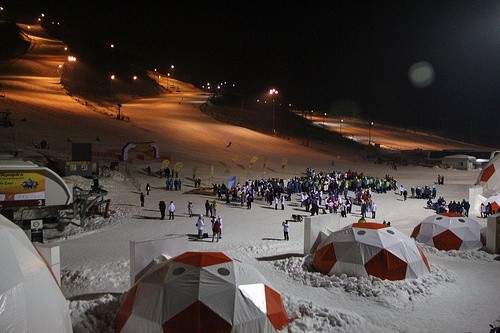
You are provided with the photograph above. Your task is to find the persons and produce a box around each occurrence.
[282,220,289,240]
[41,139,47,149]
[480,202,492,219]
[147,166,182,191]
[186,199,223,242]
[438,174,444,185]
[211,168,398,219]
[146,183,150,195]
[400,184,470,217]
[140,192,145,207]
[159,200,176,220]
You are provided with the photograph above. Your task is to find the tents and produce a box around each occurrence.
[476,152,500,198]
[114,252,289,333]
[311,222,431,281]
[0,213,74,333]
[410,212,482,251]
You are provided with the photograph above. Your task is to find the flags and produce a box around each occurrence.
[160,154,289,180]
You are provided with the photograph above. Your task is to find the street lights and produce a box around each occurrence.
[368,122,373,145]
[310,110,313,121]
[269,88,279,135]
[339,118,343,134]
[322,112,327,129]
